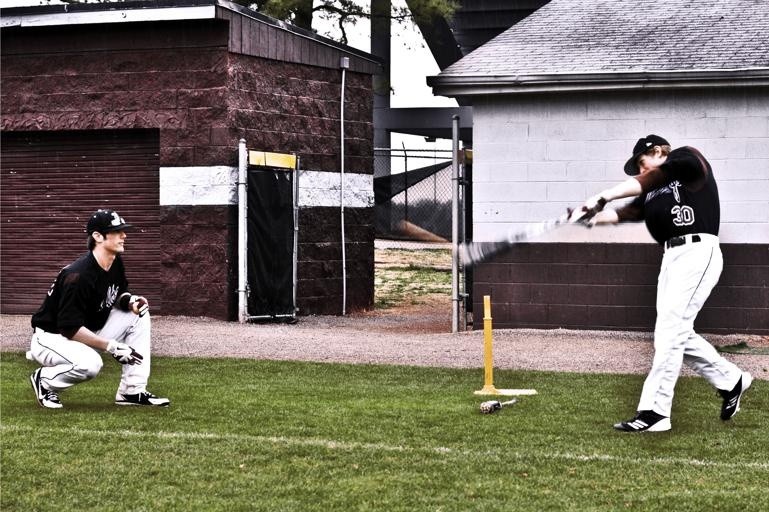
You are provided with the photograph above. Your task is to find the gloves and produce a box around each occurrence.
[106,340,144,365]
[128,296,149,318]
[567,194,607,230]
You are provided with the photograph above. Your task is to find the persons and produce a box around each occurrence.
[568,134,755,433]
[29,208,170,409]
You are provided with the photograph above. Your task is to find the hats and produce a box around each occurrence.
[87,210,133,235]
[624,134,670,176]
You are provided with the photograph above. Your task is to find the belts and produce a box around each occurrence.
[667,236,701,249]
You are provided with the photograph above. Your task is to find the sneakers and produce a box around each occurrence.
[29,366,64,409]
[115,391,170,406]
[613,410,672,432]
[718,372,752,420]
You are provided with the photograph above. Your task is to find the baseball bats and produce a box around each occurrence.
[479,397,515,414]
[454,202,583,269]
